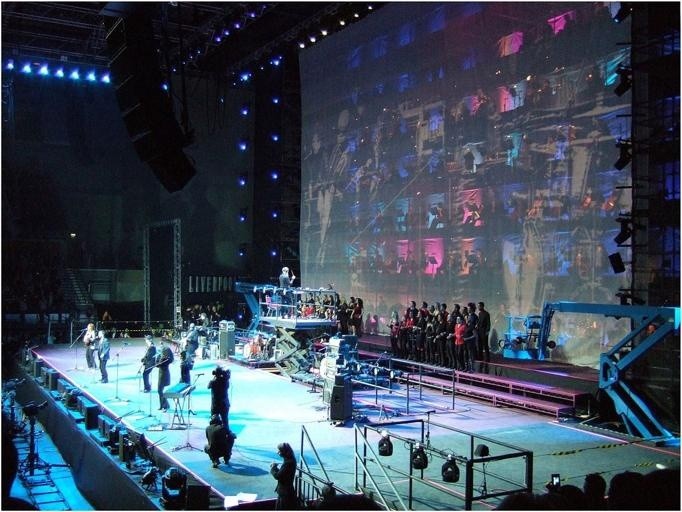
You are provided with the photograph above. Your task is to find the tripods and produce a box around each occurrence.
[65,336,86,373]
[136,370,158,420]
[172,394,204,453]
[104,361,131,404]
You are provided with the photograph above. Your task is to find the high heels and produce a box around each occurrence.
[187,408,197,417]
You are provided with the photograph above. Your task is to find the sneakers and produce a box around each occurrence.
[157,406,171,414]
[98,377,108,384]
[210,458,231,470]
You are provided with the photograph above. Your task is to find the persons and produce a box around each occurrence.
[1,267,679,511]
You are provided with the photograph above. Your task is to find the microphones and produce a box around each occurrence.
[80,328,89,333]
[123,341,132,347]
[194,372,205,377]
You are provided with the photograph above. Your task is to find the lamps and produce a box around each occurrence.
[608,3,649,308]
[355,363,404,382]
[373,428,462,482]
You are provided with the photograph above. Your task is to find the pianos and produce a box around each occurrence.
[163,382,196,398]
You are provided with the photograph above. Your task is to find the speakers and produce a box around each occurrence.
[331,385,354,419]
[96,12,198,194]
[218,330,235,360]
